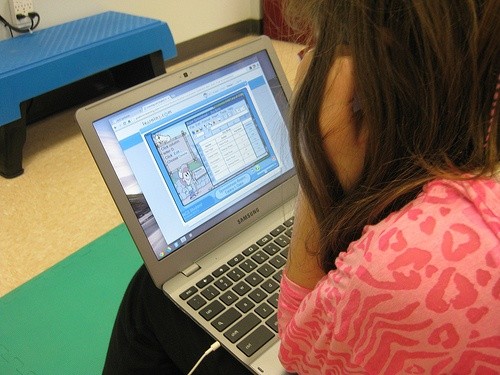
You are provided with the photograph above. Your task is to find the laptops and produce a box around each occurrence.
[74,34,301,375]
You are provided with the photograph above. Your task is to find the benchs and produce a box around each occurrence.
[0,10,179,178]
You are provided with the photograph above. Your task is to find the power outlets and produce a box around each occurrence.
[8,0,34,29]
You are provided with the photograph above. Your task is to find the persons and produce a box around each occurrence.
[101,0,500,375]
